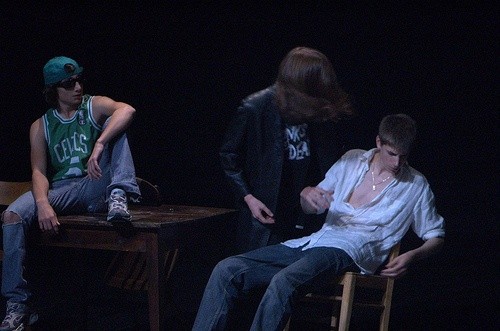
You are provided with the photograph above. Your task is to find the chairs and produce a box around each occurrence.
[278,241,401,331]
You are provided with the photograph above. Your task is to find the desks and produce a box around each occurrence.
[32,206,239,331]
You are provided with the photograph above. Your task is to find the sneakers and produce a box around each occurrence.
[0,301,38,331]
[105,191,132,221]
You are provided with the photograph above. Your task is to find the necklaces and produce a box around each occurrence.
[371,160,399,191]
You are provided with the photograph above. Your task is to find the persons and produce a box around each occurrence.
[222,47,355,254]
[192,114,445,331]
[0,55,142,331]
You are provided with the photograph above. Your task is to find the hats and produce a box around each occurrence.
[43,56,84,89]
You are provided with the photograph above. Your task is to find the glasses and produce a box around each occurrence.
[54,75,85,90]
